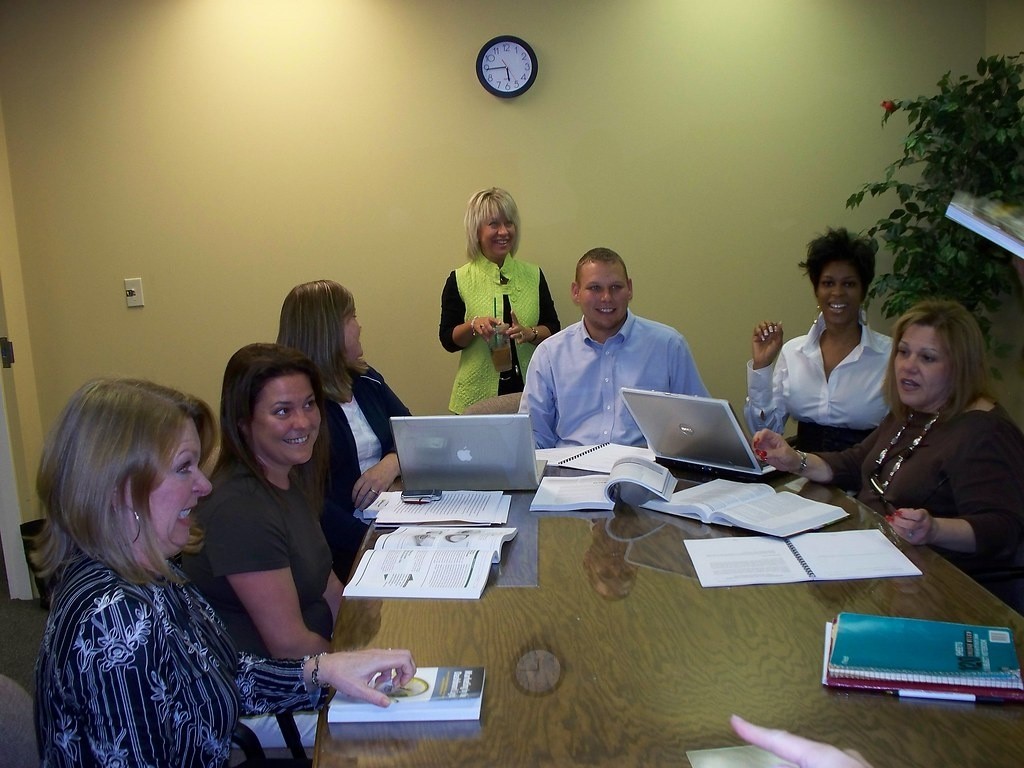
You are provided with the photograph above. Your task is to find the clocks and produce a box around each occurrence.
[475,35,538,99]
[510,643,565,697]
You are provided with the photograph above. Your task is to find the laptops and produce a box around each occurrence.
[389,414,548,491]
[619,386,778,482]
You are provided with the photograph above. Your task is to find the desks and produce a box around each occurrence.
[311,466,1024,768]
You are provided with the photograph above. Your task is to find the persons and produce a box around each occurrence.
[273,278,416,581]
[437,188,561,419]
[745,226,897,453]
[517,245,714,456]
[581,515,644,600]
[185,341,346,749]
[729,713,873,768]
[752,299,1023,604]
[19,380,414,768]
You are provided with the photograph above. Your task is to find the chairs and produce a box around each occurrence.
[0,675,44,768]
[463,392,521,415]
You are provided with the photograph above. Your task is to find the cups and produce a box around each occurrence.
[486,323,512,373]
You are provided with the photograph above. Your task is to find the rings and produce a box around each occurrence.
[370,488,379,494]
[519,332,523,336]
[480,324,484,329]
[908,531,913,539]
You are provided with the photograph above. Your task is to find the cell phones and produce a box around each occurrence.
[401,490,442,501]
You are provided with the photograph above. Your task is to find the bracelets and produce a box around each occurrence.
[311,652,332,688]
[529,326,538,342]
[793,449,807,475]
[471,315,480,336]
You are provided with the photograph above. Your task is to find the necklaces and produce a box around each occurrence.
[868,401,944,500]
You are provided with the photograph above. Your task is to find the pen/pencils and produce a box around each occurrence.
[886,688,1003,702]
[880,495,896,515]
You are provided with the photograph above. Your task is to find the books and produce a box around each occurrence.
[682,526,926,589]
[535,442,659,476]
[528,456,677,513]
[816,612,1023,703]
[637,477,851,539]
[327,660,487,723]
[341,524,518,599]
